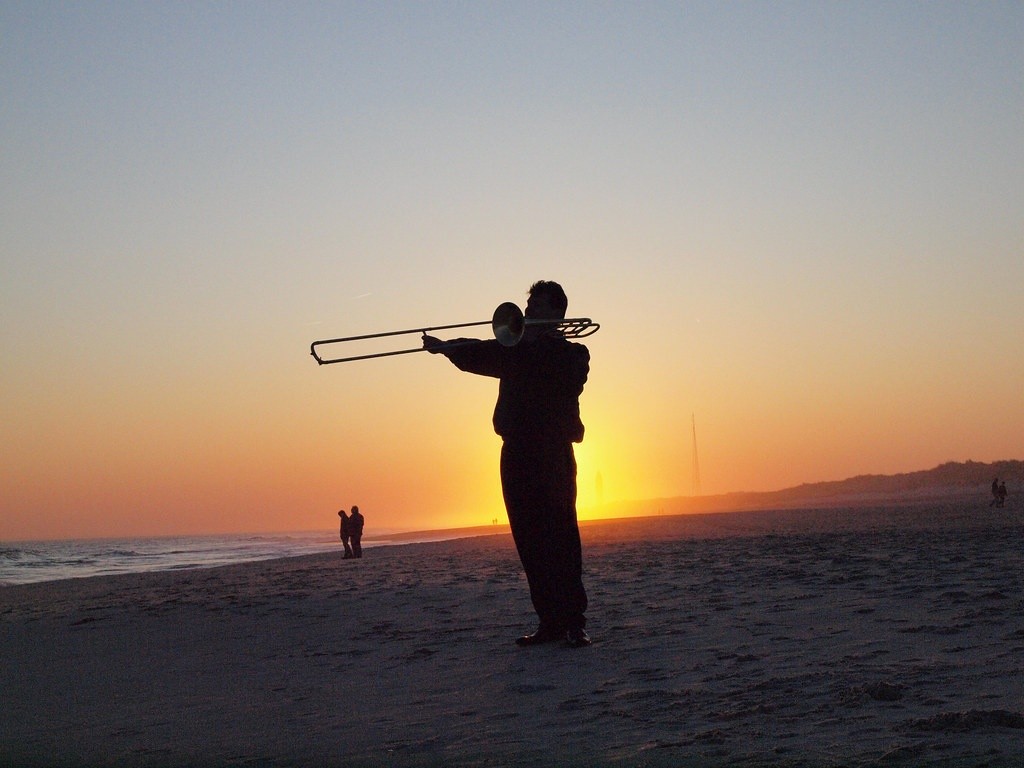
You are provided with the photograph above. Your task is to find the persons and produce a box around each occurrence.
[989,477,1008,509]
[338,506,365,559]
[422,280,591,646]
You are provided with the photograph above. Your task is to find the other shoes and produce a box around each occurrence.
[567,627,590,649]
[515,626,567,645]
[341,555,352,560]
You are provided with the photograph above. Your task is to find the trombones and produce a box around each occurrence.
[308,302,601,366]
